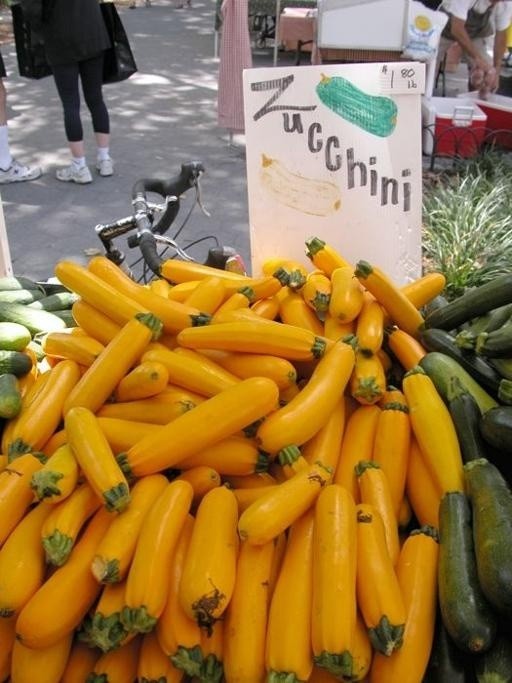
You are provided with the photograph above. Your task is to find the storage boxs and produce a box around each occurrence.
[425,89,512,160]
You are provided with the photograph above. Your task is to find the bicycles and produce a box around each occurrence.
[94,158,248,286]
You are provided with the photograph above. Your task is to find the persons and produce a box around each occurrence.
[9,0,137,184]
[435,0,512,92]
[0,50,42,183]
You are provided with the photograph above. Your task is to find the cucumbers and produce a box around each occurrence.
[261,159,340,216]
[316,77,397,137]
[0,236,512,683]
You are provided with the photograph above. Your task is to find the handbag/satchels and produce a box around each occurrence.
[99,2,137,83]
[440,5,493,40]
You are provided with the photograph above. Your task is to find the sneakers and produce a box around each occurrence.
[1,157,41,184]
[56,165,92,185]
[96,154,115,177]
